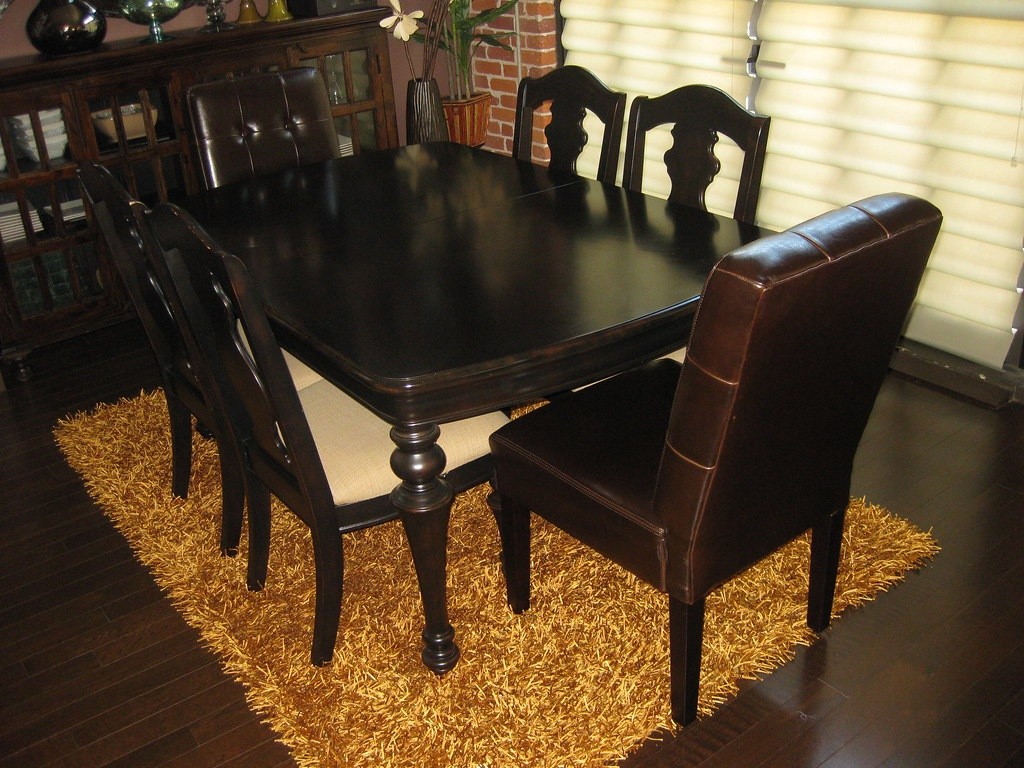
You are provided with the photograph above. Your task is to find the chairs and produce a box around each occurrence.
[78,67,942,726]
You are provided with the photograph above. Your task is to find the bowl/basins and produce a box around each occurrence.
[10,107,69,162]
[90,102,158,143]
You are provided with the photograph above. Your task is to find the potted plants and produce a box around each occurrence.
[410,0,521,149]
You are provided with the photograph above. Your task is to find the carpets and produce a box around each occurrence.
[52,386,942,768]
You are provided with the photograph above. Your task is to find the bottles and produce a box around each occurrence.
[25,0,107,58]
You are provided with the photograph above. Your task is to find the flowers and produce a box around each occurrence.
[380,0,453,80]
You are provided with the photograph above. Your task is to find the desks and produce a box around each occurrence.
[164,141,778,677]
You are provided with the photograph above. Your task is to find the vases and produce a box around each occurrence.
[407,78,448,145]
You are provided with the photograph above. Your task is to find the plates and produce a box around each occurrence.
[0,199,45,243]
[337,134,353,158]
[43,198,86,221]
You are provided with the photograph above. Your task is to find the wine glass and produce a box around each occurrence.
[117,0,185,45]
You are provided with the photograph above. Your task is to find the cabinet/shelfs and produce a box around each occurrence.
[0,9,399,382]
